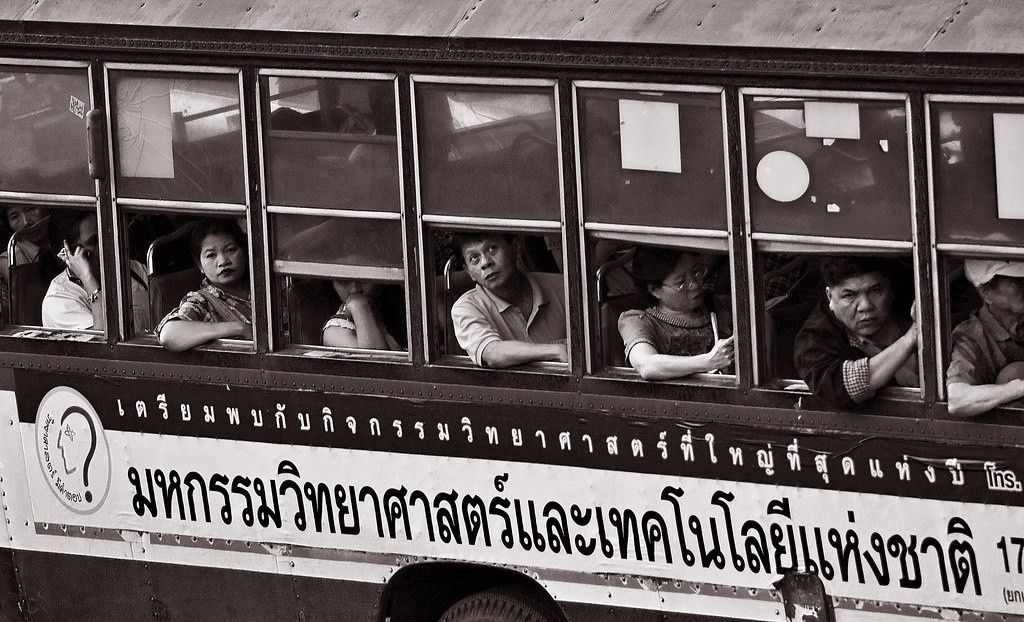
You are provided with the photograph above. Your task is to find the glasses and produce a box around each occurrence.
[659,266,707,292]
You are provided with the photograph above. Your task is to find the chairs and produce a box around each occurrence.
[0,101,981,378]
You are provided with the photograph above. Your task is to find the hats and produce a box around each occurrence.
[964,232,1024,288]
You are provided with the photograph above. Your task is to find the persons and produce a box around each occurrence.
[617,243,735,382]
[450,232,567,370]
[946,259,1024,418]
[319,239,408,352]
[793,255,918,407]
[153,218,253,353]
[0,202,159,340]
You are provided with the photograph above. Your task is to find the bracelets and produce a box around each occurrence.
[86,285,100,305]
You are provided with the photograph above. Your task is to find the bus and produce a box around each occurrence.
[0,0,1024,622]
